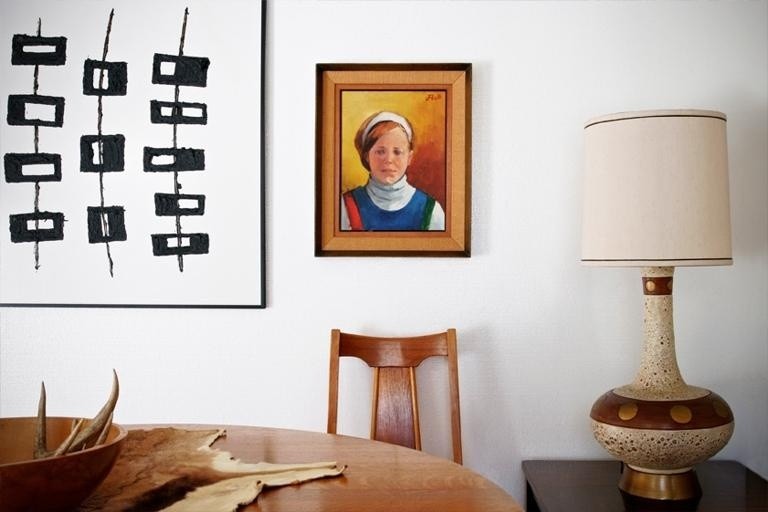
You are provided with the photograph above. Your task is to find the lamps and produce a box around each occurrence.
[578,109,737,510]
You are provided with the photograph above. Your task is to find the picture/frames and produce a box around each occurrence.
[0,0,275,313]
[311,60,472,260]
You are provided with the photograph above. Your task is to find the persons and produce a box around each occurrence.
[341,110,445,230]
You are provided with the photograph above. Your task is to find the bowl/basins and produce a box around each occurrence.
[0,417,131,511]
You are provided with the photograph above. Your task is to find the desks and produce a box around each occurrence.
[76,423,523,512]
[523,454,766,512]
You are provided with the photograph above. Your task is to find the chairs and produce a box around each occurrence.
[327,320,466,466]
